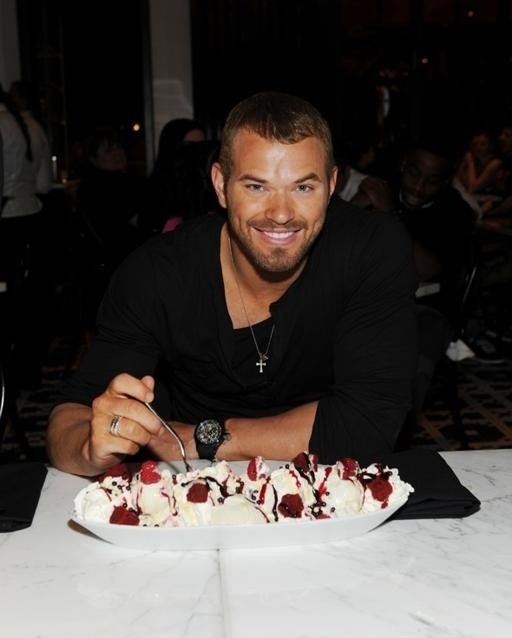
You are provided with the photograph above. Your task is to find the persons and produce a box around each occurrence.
[43,87,423,477]
[329,87,511,364]
[1,76,208,374]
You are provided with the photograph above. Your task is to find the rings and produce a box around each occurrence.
[110,416,124,433]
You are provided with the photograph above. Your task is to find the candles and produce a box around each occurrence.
[51,155,58,177]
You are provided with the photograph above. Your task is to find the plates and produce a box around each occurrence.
[69,459,410,551]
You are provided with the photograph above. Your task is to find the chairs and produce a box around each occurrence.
[393,262,481,450]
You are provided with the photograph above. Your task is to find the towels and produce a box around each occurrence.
[358,438,482,520]
[0,444,48,533]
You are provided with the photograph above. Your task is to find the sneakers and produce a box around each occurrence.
[468,336,505,365]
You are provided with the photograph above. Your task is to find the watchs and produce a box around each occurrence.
[193,415,234,461]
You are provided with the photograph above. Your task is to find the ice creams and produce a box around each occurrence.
[84,453,415,528]
